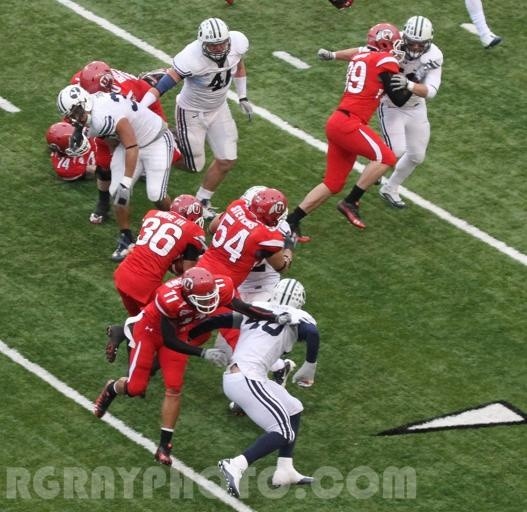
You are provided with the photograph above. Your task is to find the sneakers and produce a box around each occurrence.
[480,32,502,49]
[89,204,110,225]
[229,402,246,416]
[273,310,292,325]
[112,233,137,262]
[272,359,297,388]
[218,458,242,498]
[153,442,173,464]
[94,379,118,419]
[200,199,221,221]
[336,197,368,229]
[106,326,126,363]
[377,182,408,209]
[272,469,315,488]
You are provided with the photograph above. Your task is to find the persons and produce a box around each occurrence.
[461,1,503,52]
[45,14,446,502]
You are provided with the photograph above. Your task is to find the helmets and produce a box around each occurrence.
[197,18,231,61]
[367,23,406,63]
[403,16,434,61]
[47,60,115,158]
[170,194,205,229]
[270,278,306,310]
[182,267,220,314]
[240,185,289,230]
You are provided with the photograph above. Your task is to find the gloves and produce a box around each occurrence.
[292,359,317,388]
[390,74,408,92]
[282,231,298,254]
[239,97,253,123]
[201,347,232,368]
[317,48,336,62]
